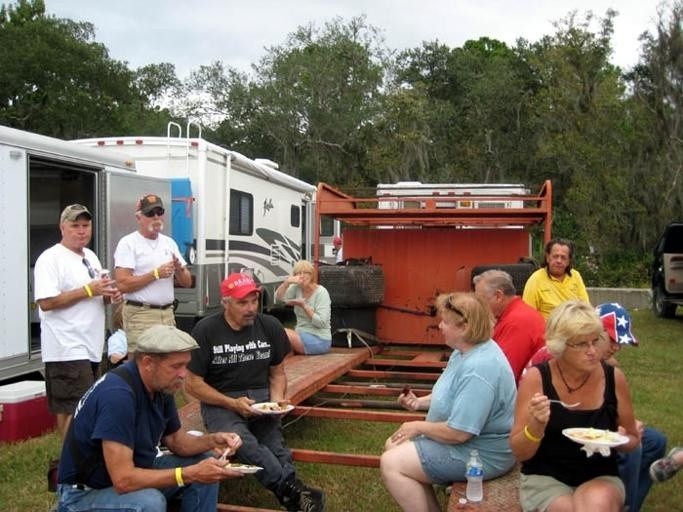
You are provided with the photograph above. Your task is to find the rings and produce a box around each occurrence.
[397,433,400,438]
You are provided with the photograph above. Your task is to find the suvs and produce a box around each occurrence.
[650,222,683,320]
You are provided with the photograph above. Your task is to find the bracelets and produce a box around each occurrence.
[174,466,184,489]
[83,284,93,297]
[524,424,542,443]
[153,268,160,281]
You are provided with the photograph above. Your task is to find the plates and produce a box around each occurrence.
[561,427,630,448]
[250,402,295,414]
[222,463,265,473]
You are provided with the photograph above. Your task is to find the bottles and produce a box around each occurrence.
[464,449,483,503]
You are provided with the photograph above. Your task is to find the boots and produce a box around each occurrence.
[269,472,326,512]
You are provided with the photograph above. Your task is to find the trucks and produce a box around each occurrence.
[62,120,344,344]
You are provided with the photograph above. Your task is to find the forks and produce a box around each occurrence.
[548,399,581,408]
[218,436,240,461]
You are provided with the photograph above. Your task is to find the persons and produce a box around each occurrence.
[379,291,519,512]
[334,238,344,266]
[517,302,666,512]
[508,302,640,512]
[113,194,191,353]
[106,303,129,367]
[523,238,589,325]
[184,272,327,512]
[471,268,547,387]
[33,202,122,442]
[56,324,243,512]
[276,259,331,355]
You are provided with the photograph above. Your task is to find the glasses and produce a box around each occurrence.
[83,258,95,279]
[445,296,467,323]
[565,333,607,350]
[144,208,165,217]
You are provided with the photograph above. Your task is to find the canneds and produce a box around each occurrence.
[100,271,112,289]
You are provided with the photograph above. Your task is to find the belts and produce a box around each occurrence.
[127,300,172,310]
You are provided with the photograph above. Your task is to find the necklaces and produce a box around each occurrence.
[556,356,592,397]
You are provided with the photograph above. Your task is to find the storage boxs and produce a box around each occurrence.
[0,380,58,443]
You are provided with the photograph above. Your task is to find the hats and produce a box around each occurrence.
[137,195,163,213]
[60,204,92,224]
[136,325,200,354]
[594,302,640,347]
[221,273,262,299]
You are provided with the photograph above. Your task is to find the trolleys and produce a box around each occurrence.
[170,176,197,266]
